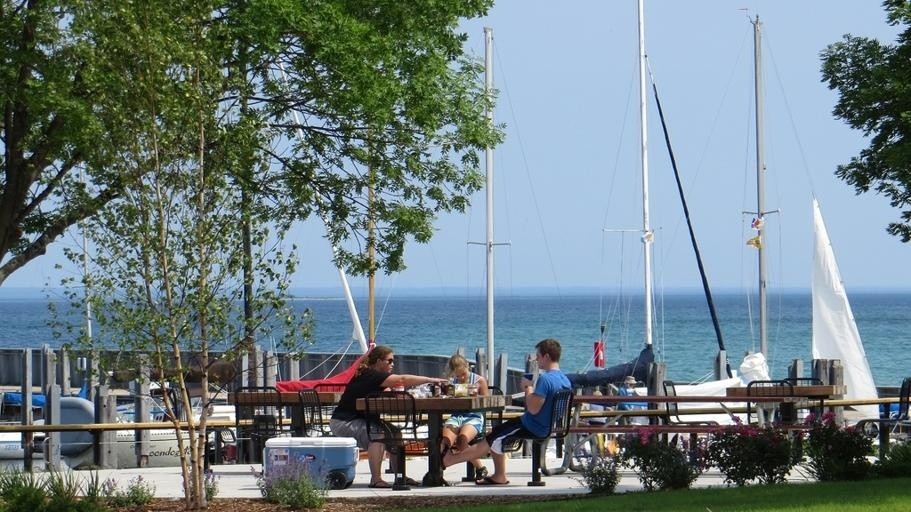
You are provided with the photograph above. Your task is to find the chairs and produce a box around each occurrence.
[785,378,824,418]
[856,377,911,465]
[235,386,296,475]
[299,389,367,453]
[747,380,807,466]
[502,389,575,486]
[663,380,722,465]
[310,383,348,434]
[171,383,236,472]
[462,386,505,482]
[365,391,430,490]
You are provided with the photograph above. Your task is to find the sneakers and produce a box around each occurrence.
[476,466,488,480]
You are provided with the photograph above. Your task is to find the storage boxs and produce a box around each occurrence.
[262,437,360,489]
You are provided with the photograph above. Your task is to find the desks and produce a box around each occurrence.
[356,394,506,486]
[726,385,847,462]
[227,391,344,438]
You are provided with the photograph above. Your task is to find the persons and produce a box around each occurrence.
[329,346,449,488]
[442,356,489,485]
[588,375,644,424]
[475,338,571,486]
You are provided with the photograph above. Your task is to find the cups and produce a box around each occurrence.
[523,373,533,385]
[467,385,478,397]
[394,383,404,393]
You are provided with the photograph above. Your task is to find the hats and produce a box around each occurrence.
[625,376,637,384]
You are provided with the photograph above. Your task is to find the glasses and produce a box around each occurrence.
[382,358,394,364]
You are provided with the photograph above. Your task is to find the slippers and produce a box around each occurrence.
[406,477,421,486]
[368,478,390,487]
[475,476,509,485]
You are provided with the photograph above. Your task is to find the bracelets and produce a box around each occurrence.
[427,378,430,384]
